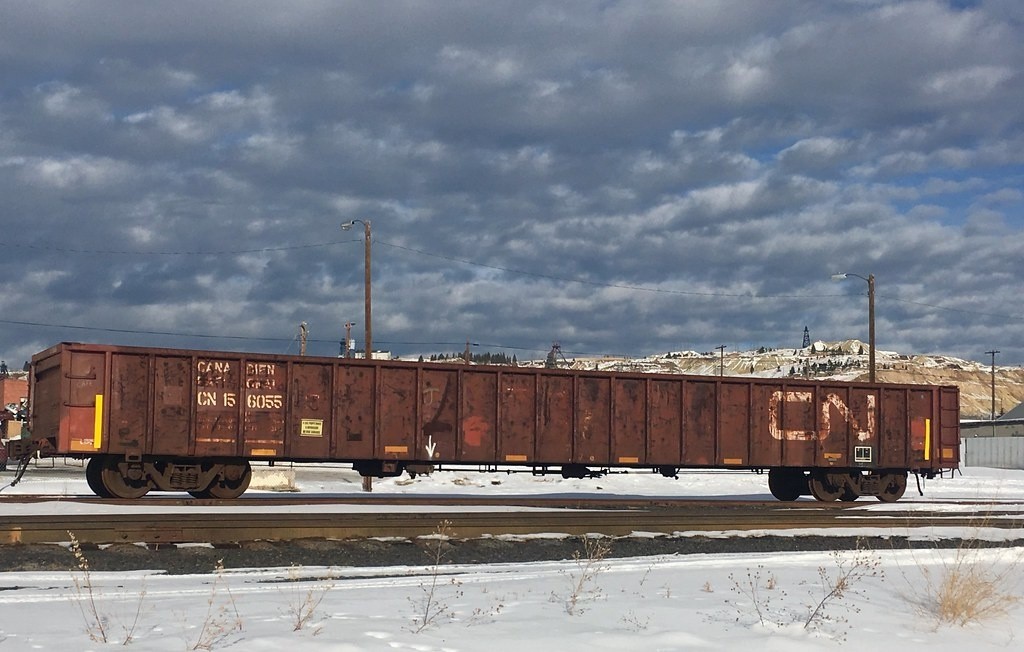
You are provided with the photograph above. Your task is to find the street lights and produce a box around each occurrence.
[832,272,876,383]
[340,216,373,359]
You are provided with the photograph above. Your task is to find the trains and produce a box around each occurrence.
[0,340,960,502]
